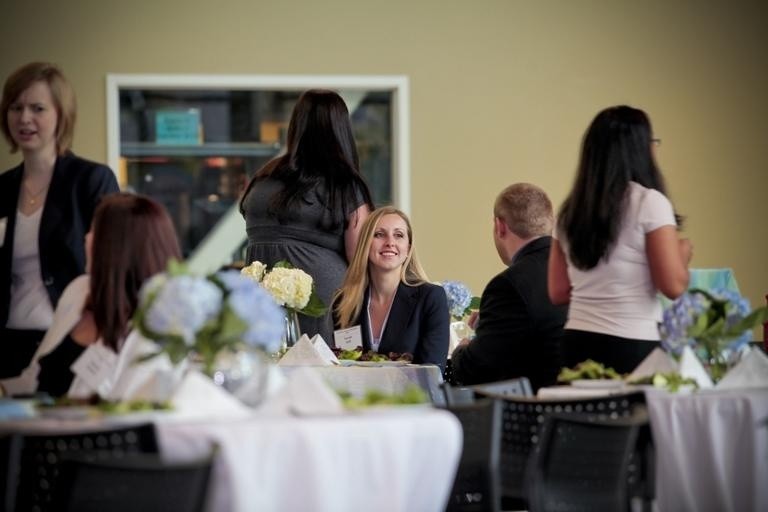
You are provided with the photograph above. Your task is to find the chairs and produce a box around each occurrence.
[533,412,647,512]
[3,420,160,509]
[58,439,217,512]
[434,403,513,512]
[473,388,656,508]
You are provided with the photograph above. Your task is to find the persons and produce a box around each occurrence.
[238,88,373,353]
[549,105,695,373]
[2,192,184,398]
[333,206,450,385]
[2,59,122,397]
[450,182,574,393]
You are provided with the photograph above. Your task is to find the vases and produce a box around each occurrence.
[282,305,300,344]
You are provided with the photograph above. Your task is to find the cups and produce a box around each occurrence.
[676,214,688,240]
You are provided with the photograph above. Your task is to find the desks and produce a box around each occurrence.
[293,354,441,399]
[539,384,767,510]
[1,390,461,511]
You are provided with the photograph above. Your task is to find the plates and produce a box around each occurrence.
[336,359,355,367]
[35,404,95,420]
[357,359,407,367]
[572,379,626,389]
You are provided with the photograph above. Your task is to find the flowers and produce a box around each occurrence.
[433,281,471,317]
[239,259,321,320]
[659,284,767,354]
[133,262,283,373]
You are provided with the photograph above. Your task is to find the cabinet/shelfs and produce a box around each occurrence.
[108,73,413,261]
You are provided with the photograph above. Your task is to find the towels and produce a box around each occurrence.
[106,330,335,406]
[630,345,764,386]
